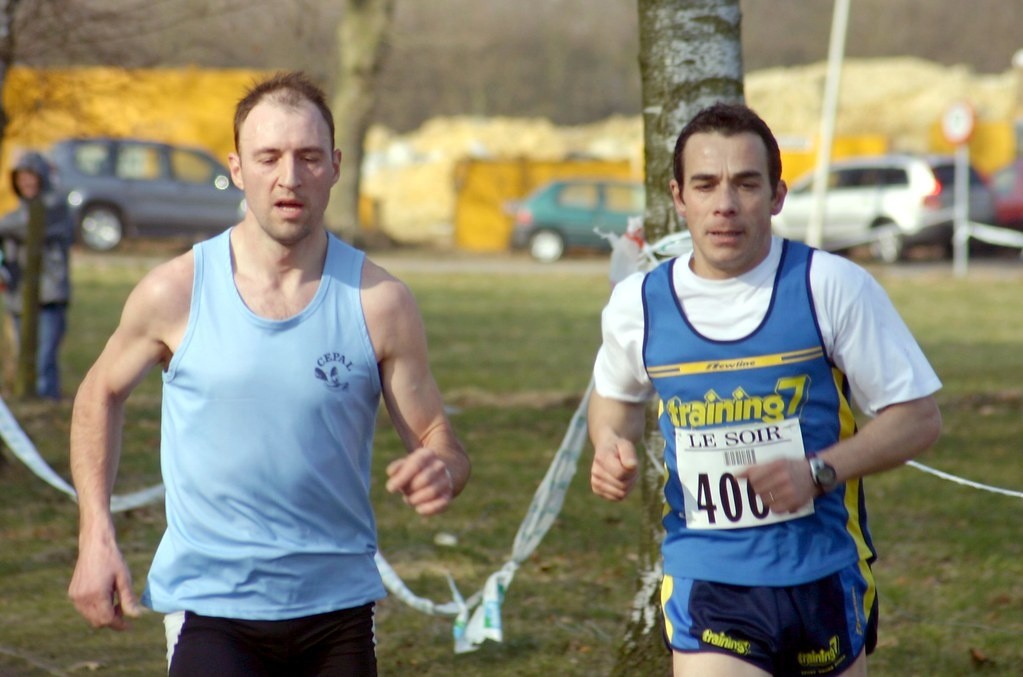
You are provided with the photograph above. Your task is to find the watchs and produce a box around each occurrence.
[805,451,837,494]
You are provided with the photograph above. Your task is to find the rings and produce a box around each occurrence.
[769,491,775,503]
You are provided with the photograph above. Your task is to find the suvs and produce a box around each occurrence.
[43,133,249,254]
[770,153,994,265]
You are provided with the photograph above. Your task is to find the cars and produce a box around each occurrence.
[941,157,1023,256]
[509,177,645,264]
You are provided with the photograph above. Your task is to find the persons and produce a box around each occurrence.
[0,153,73,401]
[589,101,943,677]
[69,71,471,676]
